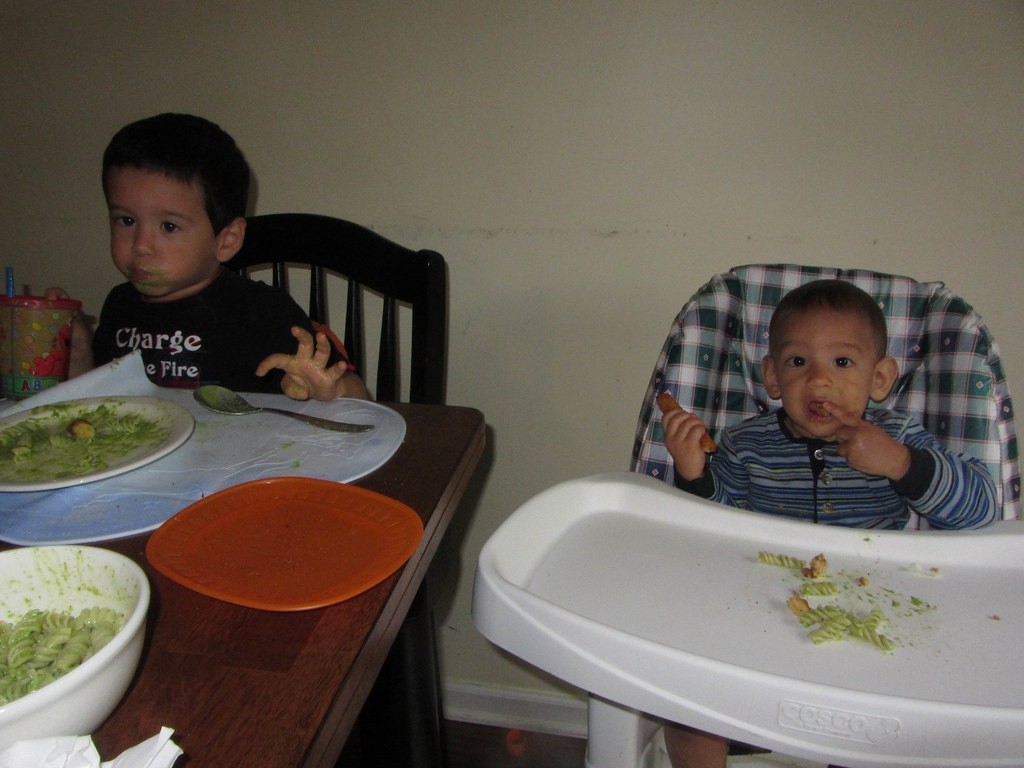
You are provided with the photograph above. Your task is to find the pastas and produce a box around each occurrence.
[759,553,893,650]
[0,406,166,469]
[0,609,118,711]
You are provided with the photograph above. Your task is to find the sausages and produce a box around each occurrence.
[657,395,716,452]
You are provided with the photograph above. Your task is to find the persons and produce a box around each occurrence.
[46,112,372,403]
[659,277,999,767]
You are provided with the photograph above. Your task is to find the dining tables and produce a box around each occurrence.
[0,388,487,768]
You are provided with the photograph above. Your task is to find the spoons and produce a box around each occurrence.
[192,385,375,434]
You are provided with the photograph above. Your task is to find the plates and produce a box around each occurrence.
[0,394,194,492]
[145,480,422,612]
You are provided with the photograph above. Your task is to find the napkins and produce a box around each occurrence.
[0,726,184,768]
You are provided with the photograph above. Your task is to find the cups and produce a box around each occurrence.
[0,294,83,401]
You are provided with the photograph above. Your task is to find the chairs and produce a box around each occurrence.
[221,213,447,408]
[472,262,1024,767]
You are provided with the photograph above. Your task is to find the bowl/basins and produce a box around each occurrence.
[0,545,151,752]
